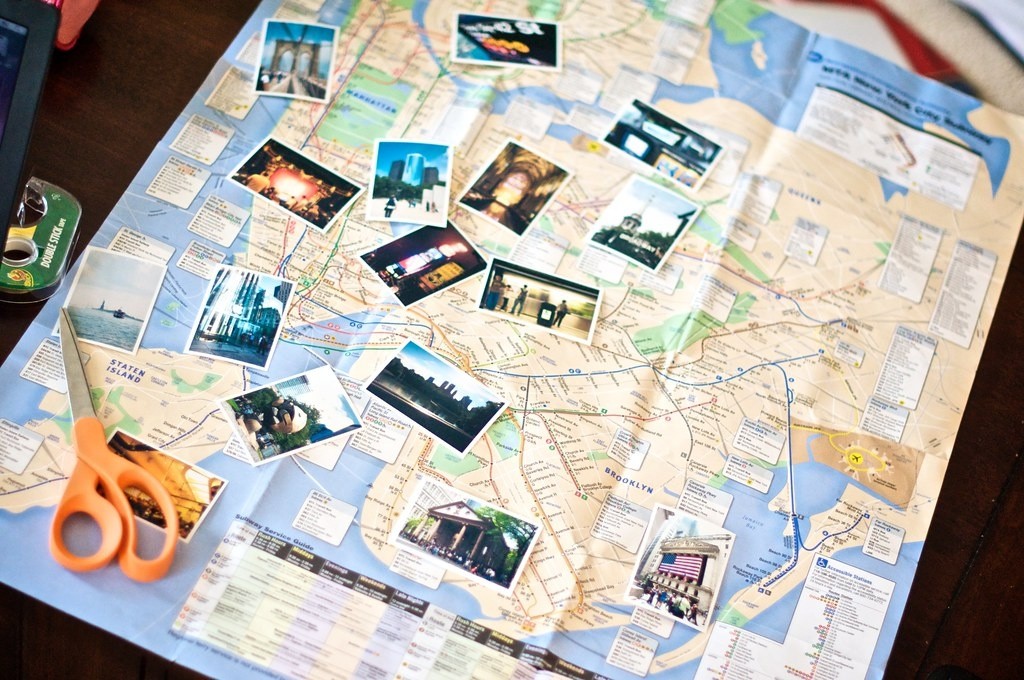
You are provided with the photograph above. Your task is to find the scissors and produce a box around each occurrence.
[50,306,178,583]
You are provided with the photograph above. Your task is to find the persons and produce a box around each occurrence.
[277,72,282,83]
[399,530,495,581]
[244,169,319,222]
[648,588,698,625]
[486,274,506,311]
[553,300,567,327]
[384,194,396,216]
[509,284,529,317]
[261,71,270,92]
[501,284,513,311]
